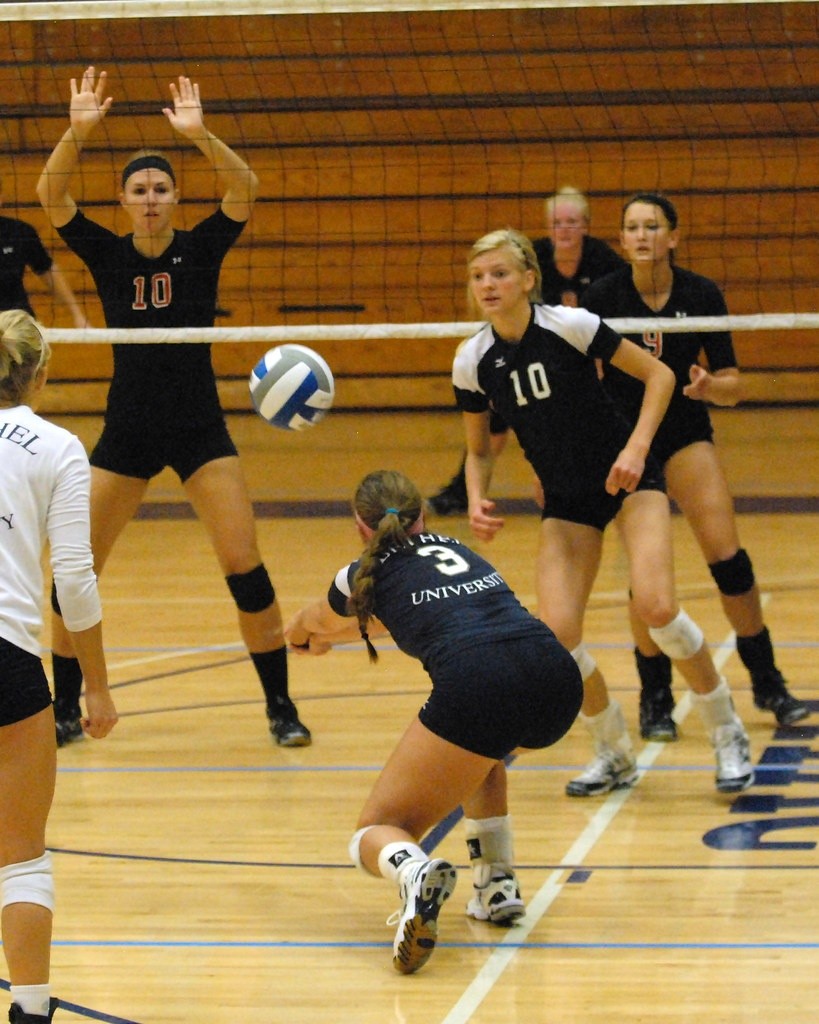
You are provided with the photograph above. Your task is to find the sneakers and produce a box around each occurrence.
[703,713,755,794]
[53,697,85,747]
[464,873,527,925]
[266,694,312,748]
[392,856,458,974]
[638,685,677,741]
[752,670,809,724]
[564,744,642,798]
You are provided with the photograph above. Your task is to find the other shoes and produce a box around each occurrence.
[8,997,60,1023]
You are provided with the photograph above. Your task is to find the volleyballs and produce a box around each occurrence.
[249,343,335,431]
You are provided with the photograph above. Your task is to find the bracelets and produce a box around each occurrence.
[290,639,311,650]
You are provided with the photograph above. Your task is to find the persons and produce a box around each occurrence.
[0,181,91,329]
[281,471,587,976]
[421,186,812,741]
[451,227,760,798]
[0,303,121,1024]
[33,66,318,748]
